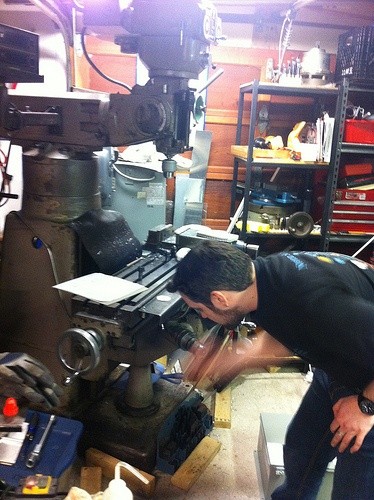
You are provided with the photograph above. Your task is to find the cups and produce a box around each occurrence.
[3,398,18,417]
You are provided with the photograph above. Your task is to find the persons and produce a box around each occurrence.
[166,239,374,500]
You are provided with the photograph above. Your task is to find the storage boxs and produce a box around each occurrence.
[333,24,374,84]
[343,118,374,143]
[0,413,83,494]
[312,159,374,234]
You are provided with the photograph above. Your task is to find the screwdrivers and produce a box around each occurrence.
[20,412,40,460]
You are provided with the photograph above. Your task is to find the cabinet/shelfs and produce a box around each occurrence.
[230,81,374,256]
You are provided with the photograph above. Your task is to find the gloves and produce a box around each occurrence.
[0,353,63,411]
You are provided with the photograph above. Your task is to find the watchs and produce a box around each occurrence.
[358,392,374,416]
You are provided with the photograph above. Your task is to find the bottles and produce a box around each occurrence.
[102,478,133,499]
